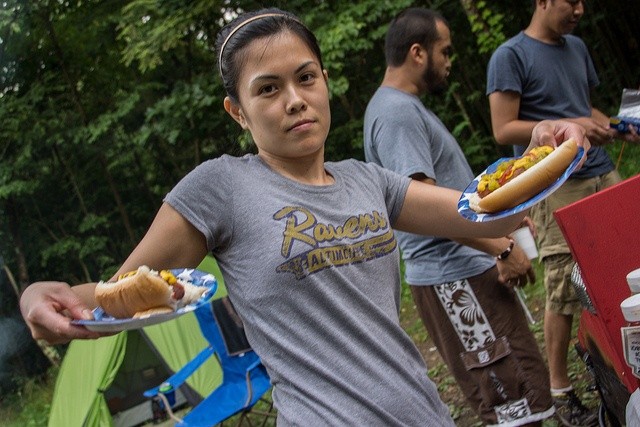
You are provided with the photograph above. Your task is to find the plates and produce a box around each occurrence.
[458,146,584,223]
[70,267,217,333]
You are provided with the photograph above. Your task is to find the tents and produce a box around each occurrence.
[47,254,226,427]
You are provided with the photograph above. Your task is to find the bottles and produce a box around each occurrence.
[620,293,640,389]
[626,268,640,296]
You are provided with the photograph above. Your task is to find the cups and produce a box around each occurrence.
[507,225,539,261]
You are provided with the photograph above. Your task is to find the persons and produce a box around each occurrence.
[364,7,557,427]
[487,1,640,427]
[20,7,592,427]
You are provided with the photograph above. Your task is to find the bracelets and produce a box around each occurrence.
[497,239,514,261]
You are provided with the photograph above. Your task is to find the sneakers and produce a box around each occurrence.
[552,387,599,427]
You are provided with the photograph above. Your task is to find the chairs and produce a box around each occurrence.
[143,295,277,427]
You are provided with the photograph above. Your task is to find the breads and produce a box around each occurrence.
[95,264,207,319]
[468,136,578,212]
